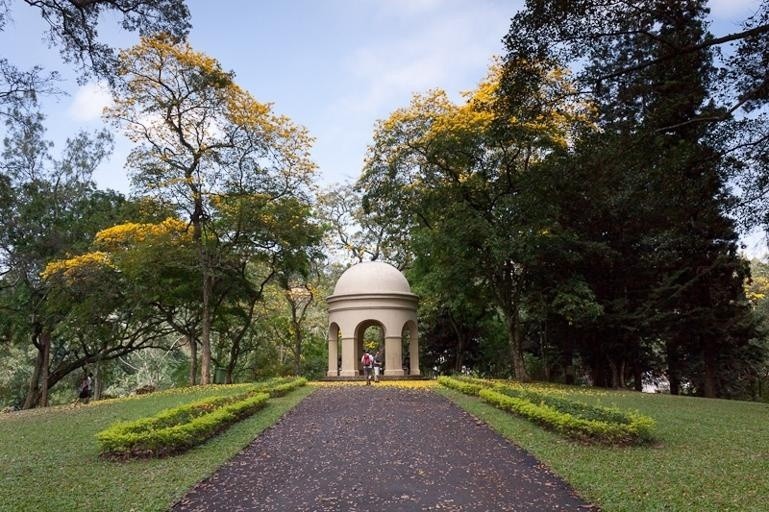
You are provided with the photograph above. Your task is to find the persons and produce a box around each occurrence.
[373,350,382,383]
[73,373,88,407]
[86,371,93,404]
[360,349,373,385]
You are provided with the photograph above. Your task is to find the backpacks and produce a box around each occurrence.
[362,354,370,366]
